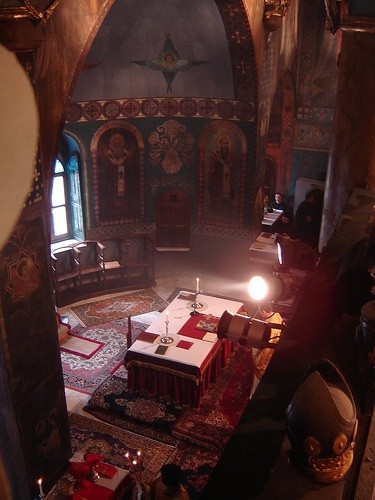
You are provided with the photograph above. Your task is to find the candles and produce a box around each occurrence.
[38,479,42,495]
[196,278,199,291]
[166,311,169,321]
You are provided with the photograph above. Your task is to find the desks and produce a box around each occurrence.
[124,291,245,410]
[44,461,130,500]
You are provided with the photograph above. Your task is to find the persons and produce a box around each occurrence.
[296,189,322,248]
[268,192,290,224]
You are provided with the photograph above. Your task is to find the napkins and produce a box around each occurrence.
[175,340,193,349]
[203,332,217,342]
[154,345,168,355]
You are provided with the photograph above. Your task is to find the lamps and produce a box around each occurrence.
[278,242,308,265]
[248,274,294,301]
[217,311,271,349]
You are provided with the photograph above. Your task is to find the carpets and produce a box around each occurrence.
[24,287,284,483]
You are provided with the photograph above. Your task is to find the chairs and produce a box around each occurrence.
[51,234,155,308]
